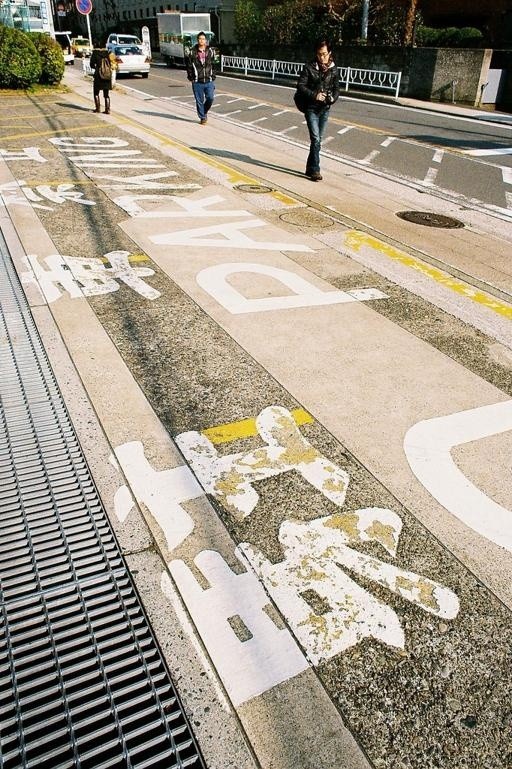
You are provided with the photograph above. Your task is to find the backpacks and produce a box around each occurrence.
[98,58,111,80]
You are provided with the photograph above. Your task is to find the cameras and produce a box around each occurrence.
[322,91,335,107]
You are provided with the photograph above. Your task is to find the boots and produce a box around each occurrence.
[94,95,110,114]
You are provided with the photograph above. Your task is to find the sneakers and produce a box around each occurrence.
[311,170,322,181]
[201,114,208,124]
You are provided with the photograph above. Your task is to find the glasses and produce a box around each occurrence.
[317,53,329,57]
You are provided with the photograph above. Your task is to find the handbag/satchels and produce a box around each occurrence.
[293,89,313,113]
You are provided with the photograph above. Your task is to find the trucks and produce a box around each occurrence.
[156,12,221,70]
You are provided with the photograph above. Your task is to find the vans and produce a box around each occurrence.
[54,30,151,78]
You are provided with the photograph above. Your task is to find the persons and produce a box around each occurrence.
[296,38,341,181]
[185,29,216,127]
[89,37,114,115]
